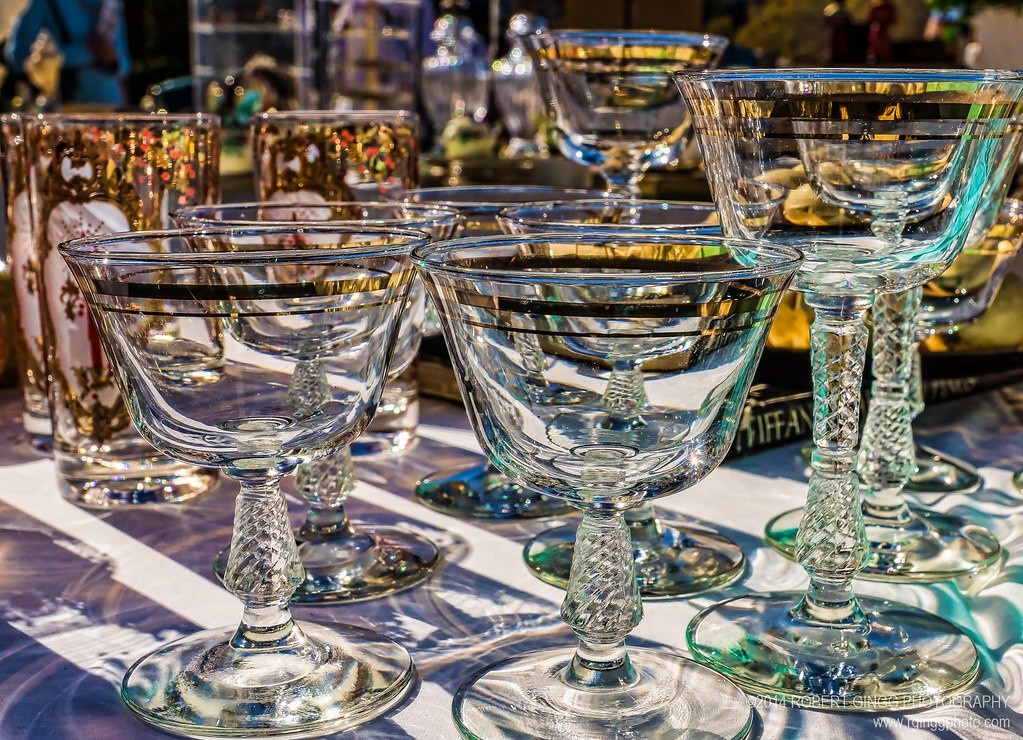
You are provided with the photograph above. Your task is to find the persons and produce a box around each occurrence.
[245,58,300,120]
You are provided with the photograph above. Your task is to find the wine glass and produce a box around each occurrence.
[51,27,1022,739]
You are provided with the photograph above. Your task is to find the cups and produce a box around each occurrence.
[1,109,223,508]
[248,108,420,431]
[188,1,429,188]
